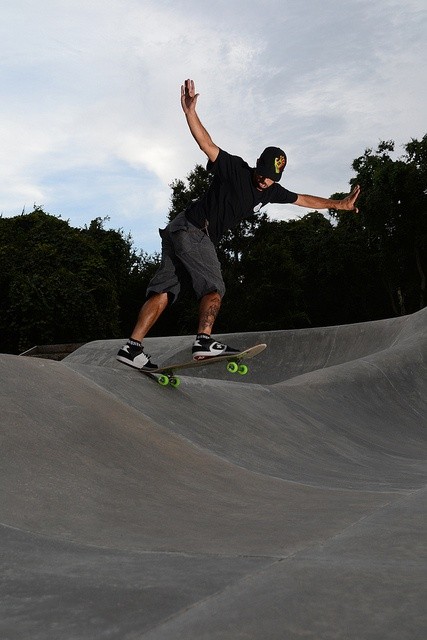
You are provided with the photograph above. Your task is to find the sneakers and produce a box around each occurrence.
[191,338,242,359]
[115,344,158,371]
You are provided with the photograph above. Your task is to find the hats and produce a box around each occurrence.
[256,146,288,183]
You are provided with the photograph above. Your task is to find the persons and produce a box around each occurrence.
[115,78,360,372]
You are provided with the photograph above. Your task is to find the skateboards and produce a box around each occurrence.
[140,344,267,387]
